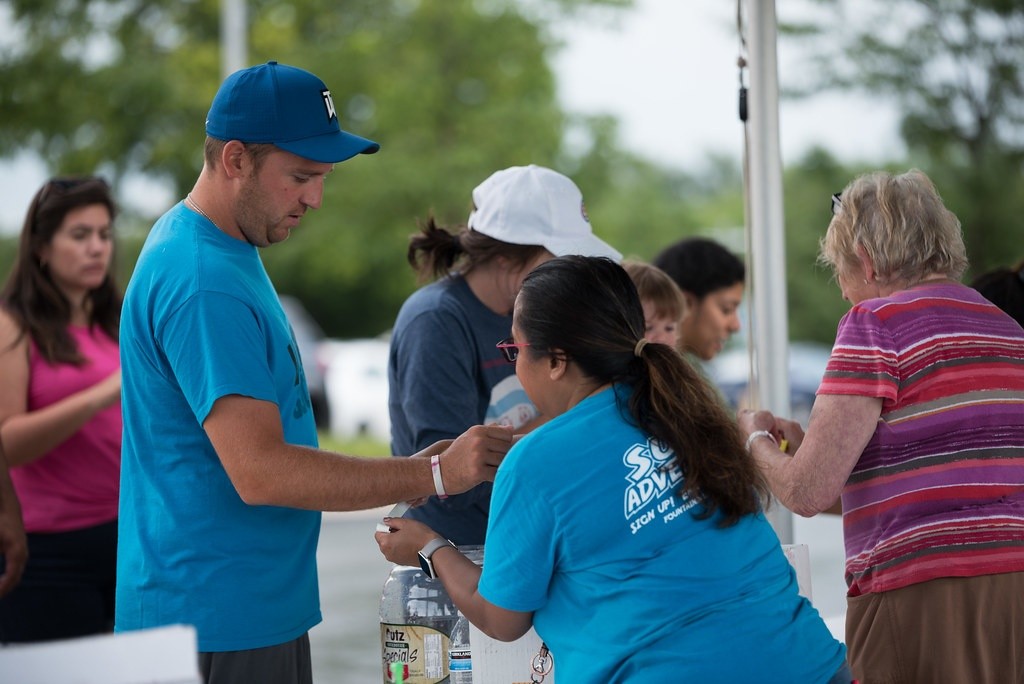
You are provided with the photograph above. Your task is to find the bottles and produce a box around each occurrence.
[377,542,484,684]
[447,609,472,684]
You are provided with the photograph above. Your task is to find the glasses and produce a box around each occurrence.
[495,338,532,363]
[830,192,848,212]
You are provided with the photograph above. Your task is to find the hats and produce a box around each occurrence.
[467,164,623,265]
[205,60,380,163]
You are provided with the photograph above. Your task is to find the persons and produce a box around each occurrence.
[385,164,621,551]
[111,59,517,684]
[0,176,125,645]
[622,256,688,351]
[376,254,854,684]
[644,236,747,414]
[738,167,1024,684]
[0,440,28,593]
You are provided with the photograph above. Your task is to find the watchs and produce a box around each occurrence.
[417,538,458,579]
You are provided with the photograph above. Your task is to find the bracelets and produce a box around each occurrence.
[431,454,448,503]
[745,431,789,454]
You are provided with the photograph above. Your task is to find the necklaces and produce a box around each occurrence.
[185,193,221,231]
